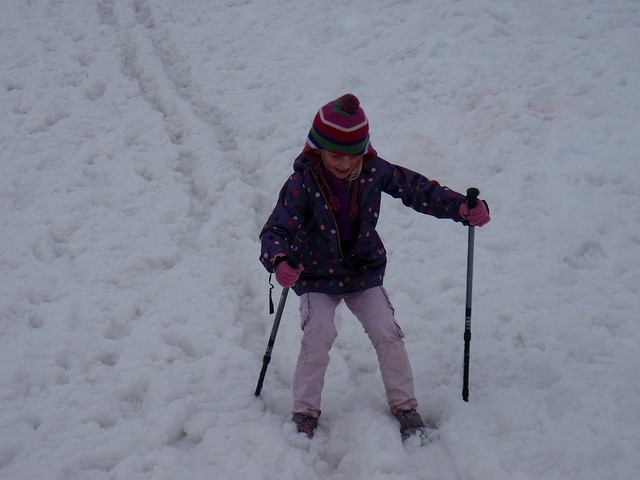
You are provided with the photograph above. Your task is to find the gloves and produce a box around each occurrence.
[272,252,303,288]
[459,200,491,227]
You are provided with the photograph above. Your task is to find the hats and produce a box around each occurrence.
[301,93,377,220]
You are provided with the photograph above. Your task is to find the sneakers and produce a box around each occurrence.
[291,409,321,440]
[395,409,428,448]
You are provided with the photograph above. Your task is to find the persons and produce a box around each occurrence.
[259,93,491,445]
